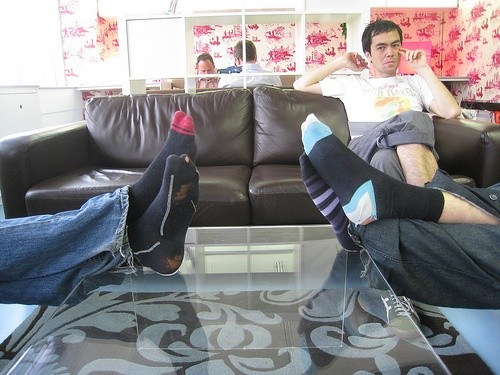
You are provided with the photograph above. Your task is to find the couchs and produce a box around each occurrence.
[0,86,500,224]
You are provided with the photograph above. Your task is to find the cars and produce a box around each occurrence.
[217,66,243,74]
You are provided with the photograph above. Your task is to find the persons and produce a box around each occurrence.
[218,40,282,89]
[196,53,220,89]
[0,111,199,306]
[293,20,461,339]
[299,113,500,311]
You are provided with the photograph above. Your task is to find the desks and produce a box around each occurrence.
[6,226,453,375]
